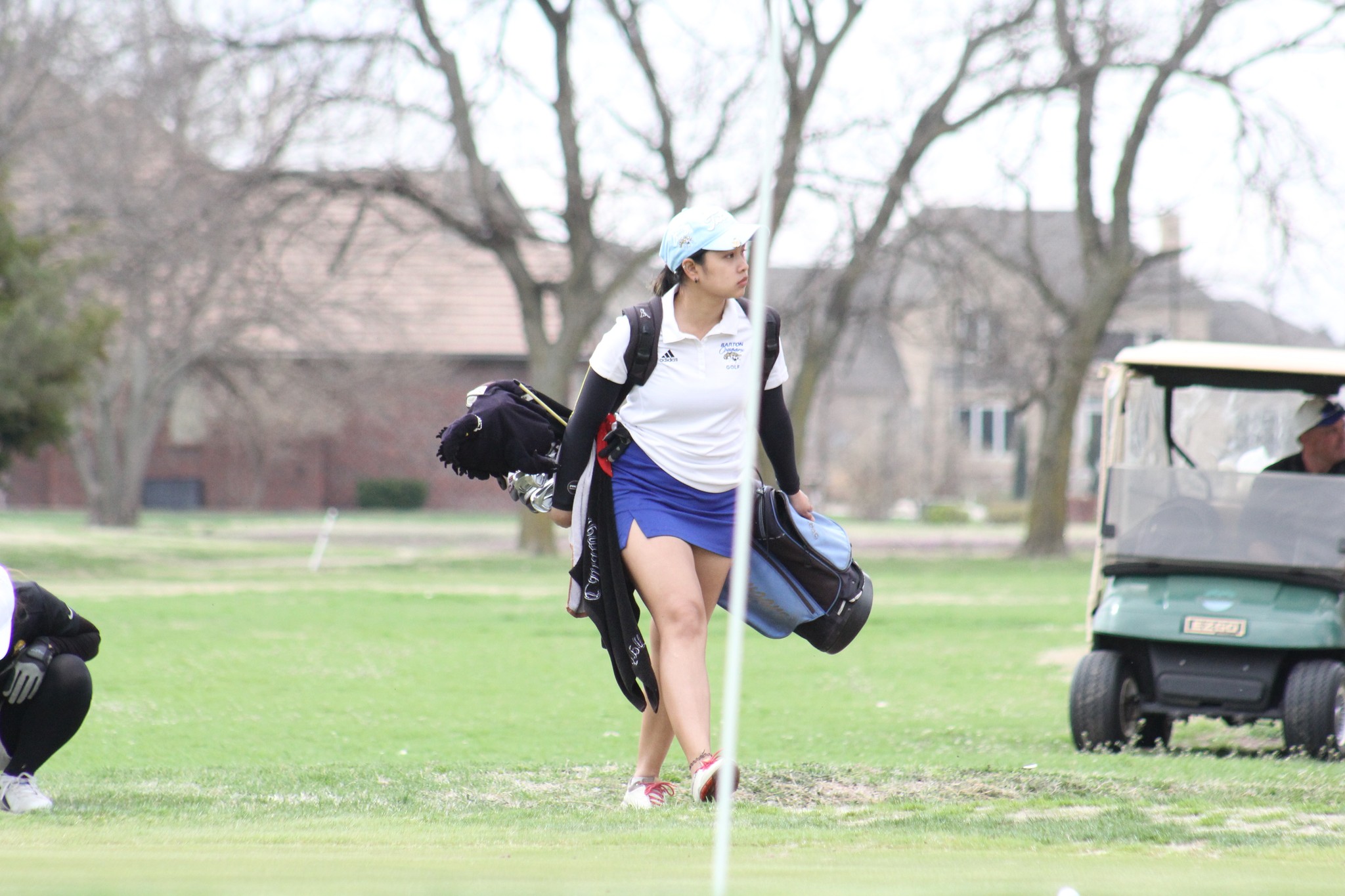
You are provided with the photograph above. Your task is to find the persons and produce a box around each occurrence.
[0,563,100,814]
[550,204,814,813]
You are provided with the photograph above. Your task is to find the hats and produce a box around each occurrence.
[0,563,16,659]
[1292,398,1345,441]
[658,203,761,274]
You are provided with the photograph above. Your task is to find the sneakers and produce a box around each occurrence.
[620,776,677,809]
[691,748,740,803]
[0,771,53,813]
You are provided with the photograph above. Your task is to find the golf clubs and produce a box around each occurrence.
[495,444,560,514]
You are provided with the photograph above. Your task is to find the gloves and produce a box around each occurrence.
[0,637,55,704]
